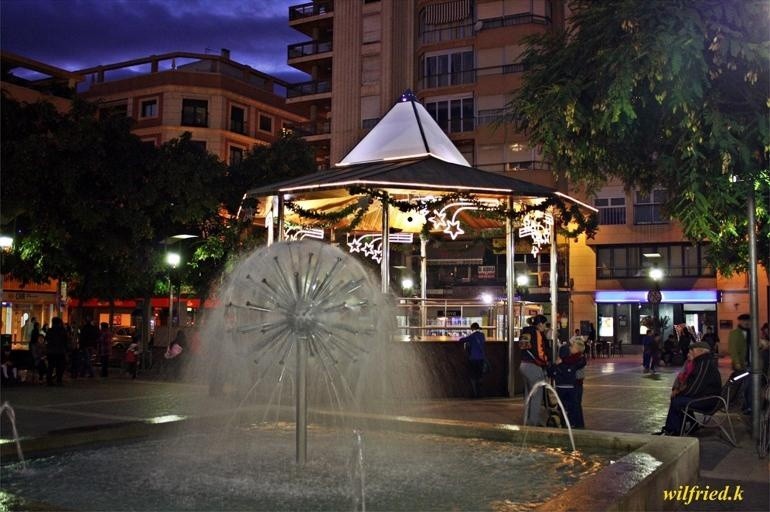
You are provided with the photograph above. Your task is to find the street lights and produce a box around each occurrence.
[644,248,664,337]
[393,261,416,337]
[0,232,16,258]
[158,230,201,378]
[515,261,533,323]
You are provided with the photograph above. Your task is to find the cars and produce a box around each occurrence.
[108,323,135,350]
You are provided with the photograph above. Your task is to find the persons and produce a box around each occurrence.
[516,313,604,431]
[0,317,193,389]
[458,322,486,400]
[642,313,769,436]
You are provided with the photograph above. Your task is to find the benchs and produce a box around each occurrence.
[680,367,752,450]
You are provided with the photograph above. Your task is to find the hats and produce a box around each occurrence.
[691,342,711,349]
[738,314,751,320]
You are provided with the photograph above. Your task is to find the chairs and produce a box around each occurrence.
[572,337,624,360]
